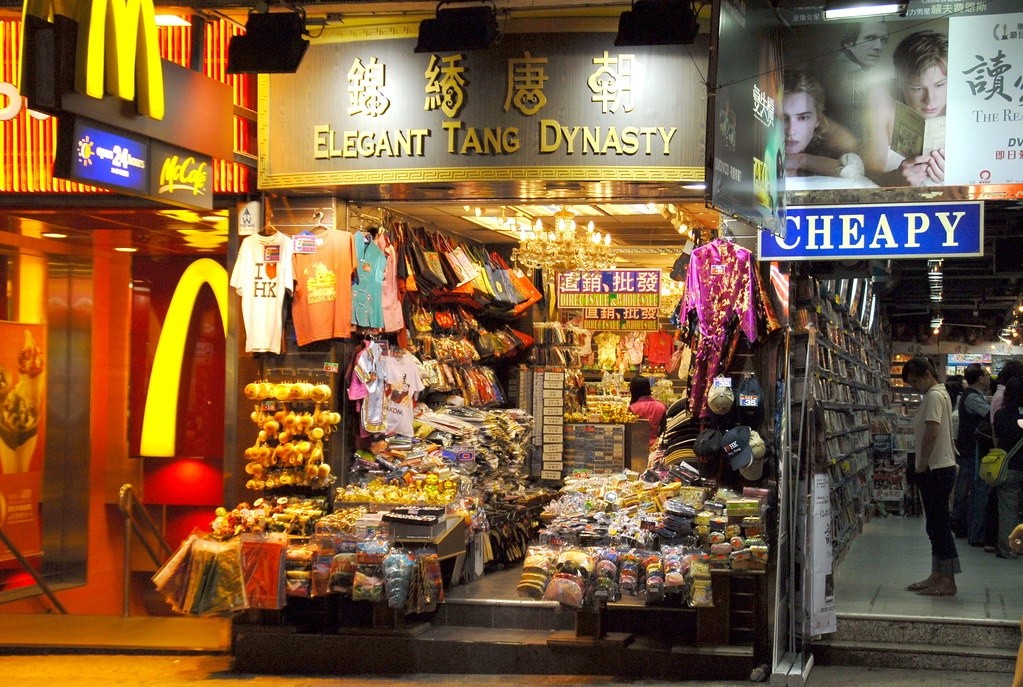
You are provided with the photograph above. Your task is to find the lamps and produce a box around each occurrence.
[614,0,700,48]
[225,11,310,75]
[463,202,704,284]
[822,0,907,21]
[926,258,1022,344]
[412,1,500,50]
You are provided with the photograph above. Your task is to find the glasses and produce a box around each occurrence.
[979,372,991,376]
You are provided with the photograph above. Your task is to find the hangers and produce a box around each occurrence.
[714,221,734,248]
[256,211,277,236]
[360,331,406,363]
[300,210,395,250]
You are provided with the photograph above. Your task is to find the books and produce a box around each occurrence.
[791,274,926,575]
[889,99,946,166]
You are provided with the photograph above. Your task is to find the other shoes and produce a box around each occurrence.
[996,548,1018,559]
[952,528,968,538]
[984,544,1004,552]
[967,539,988,547]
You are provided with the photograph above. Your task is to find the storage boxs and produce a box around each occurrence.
[616,469,770,571]
[382,501,467,561]
[872,434,913,515]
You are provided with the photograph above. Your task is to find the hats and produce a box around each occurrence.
[692,372,766,480]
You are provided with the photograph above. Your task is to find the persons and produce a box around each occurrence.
[626,375,667,448]
[902,355,1023,687]
[782,22,949,187]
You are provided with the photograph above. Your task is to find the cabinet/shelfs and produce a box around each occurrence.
[790,333,891,574]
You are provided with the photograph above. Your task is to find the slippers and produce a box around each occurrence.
[916,585,956,596]
[905,582,934,590]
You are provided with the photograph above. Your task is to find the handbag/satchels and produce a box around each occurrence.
[380,215,541,366]
[972,420,992,441]
[666,342,692,382]
[979,449,1009,486]
